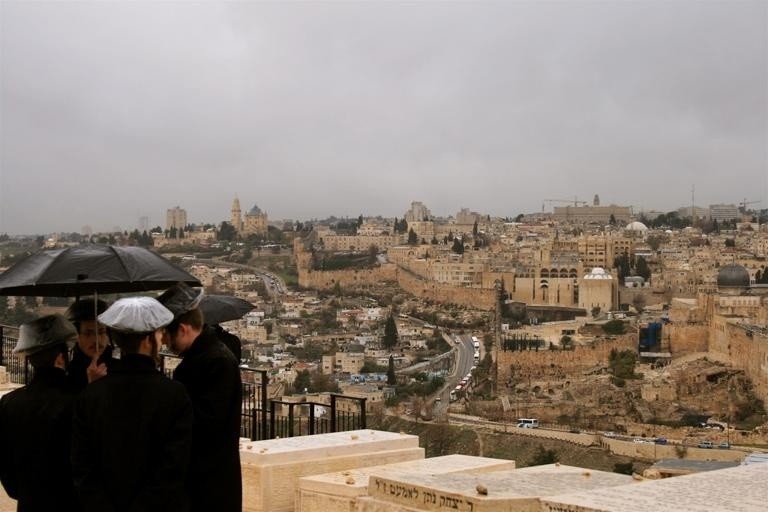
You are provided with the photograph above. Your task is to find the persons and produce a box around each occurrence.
[2,281,243,510]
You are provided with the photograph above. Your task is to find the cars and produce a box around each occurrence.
[454,339,461,344]
[604,431,616,438]
[718,443,731,449]
[632,436,668,447]
[435,395,441,402]
[259,270,285,296]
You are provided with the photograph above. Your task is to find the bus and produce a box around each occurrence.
[449,365,478,401]
[397,314,408,323]
[182,255,196,260]
[516,417,539,430]
[469,336,482,365]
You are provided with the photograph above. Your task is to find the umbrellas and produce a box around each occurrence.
[198,294,258,325]
[0,242,204,366]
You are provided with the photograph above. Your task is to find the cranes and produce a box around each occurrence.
[544,196,588,208]
[740,196,762,217]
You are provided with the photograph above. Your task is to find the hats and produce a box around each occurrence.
[97,295,176,333]
[64,298,108,320]
[157,281,205,317]
[14,314,79,356]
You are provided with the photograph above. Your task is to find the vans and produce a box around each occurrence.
[699,441,713,448]
